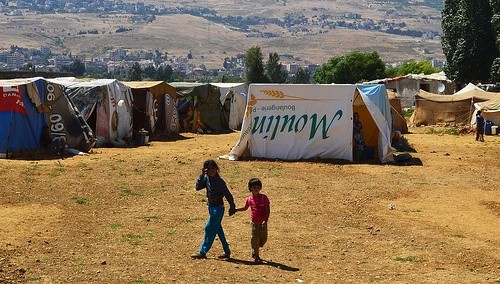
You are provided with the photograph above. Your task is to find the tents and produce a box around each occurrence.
[0,70,500,163]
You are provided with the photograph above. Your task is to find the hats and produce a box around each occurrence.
[354,112,359,117]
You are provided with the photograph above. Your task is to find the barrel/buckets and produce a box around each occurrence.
[491,125,498,134]
[144,136,149,146]
[484,121,493,135]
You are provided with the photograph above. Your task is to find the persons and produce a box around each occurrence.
[353,112,366,154]
[476,110,485,142]
[190,160,235,258]
[229,177,270,263]
[41,125,66,156]
[392,131,406,151]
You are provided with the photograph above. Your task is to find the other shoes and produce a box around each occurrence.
[255,257,260,264]
[217,254,230,260]
[190,252,207,259]
